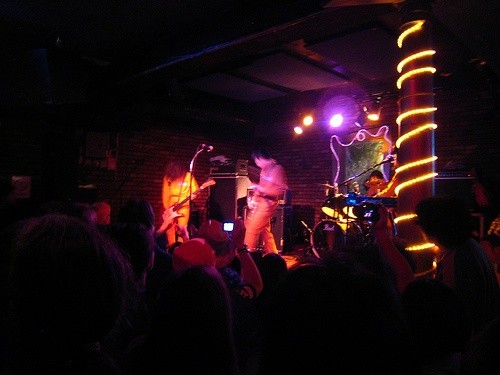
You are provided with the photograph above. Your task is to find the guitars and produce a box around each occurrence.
[161,179,216,224]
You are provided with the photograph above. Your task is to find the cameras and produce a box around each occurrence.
[352,202,381,222]
[223,222,234,232]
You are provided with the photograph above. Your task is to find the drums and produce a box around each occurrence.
[342,198,360,219]
[321,196,341,218]
[309,216,363,259]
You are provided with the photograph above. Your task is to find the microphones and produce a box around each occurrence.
[201,144,213,152]
[388,155,396,163]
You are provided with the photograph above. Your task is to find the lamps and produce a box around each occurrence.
[368,96,383,121]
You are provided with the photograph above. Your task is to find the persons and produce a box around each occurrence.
[364,169,398,245]
[162,158,200,252]
[0,183,500,375]
[243,149,288,256]
